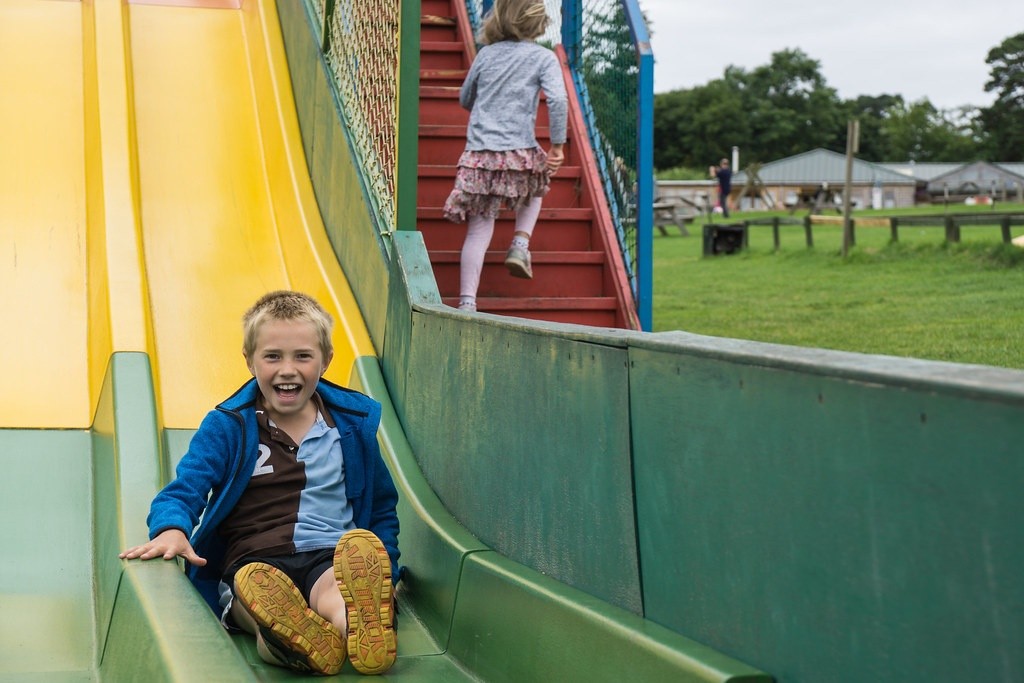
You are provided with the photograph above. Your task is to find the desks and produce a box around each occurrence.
[630,202,690,237]
[792,187,844,215]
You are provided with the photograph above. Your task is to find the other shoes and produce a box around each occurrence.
[506,246,532,279]
[334,528,399,674]
[234,562,347,676]
[459,302,476,313]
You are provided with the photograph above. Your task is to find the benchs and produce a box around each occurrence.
[659,214,695,224]
[784,198,855,209]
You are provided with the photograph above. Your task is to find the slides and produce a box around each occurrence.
[0,0,771,683]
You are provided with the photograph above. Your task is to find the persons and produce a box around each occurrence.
[708,157,732,218]
[991,180,998,200]
[943,182,951,206]
[117,290,413,675]
[444,0,568,314]
